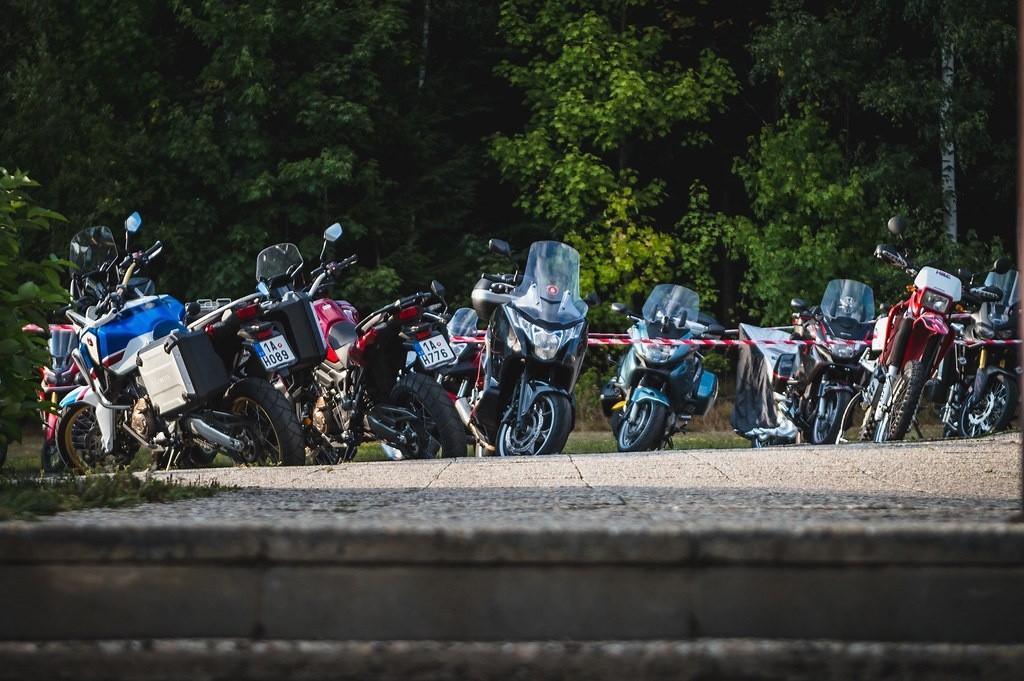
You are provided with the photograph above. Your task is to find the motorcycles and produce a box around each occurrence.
[455,238,600,459]
[857,217,1013,447]
[54,211,328,468]
[925,267,1023,439]
[383,307,486,462]
[772,279,875,446]
[253,223,467,466]
[601,284,726,453]
[21,323,86,472]
